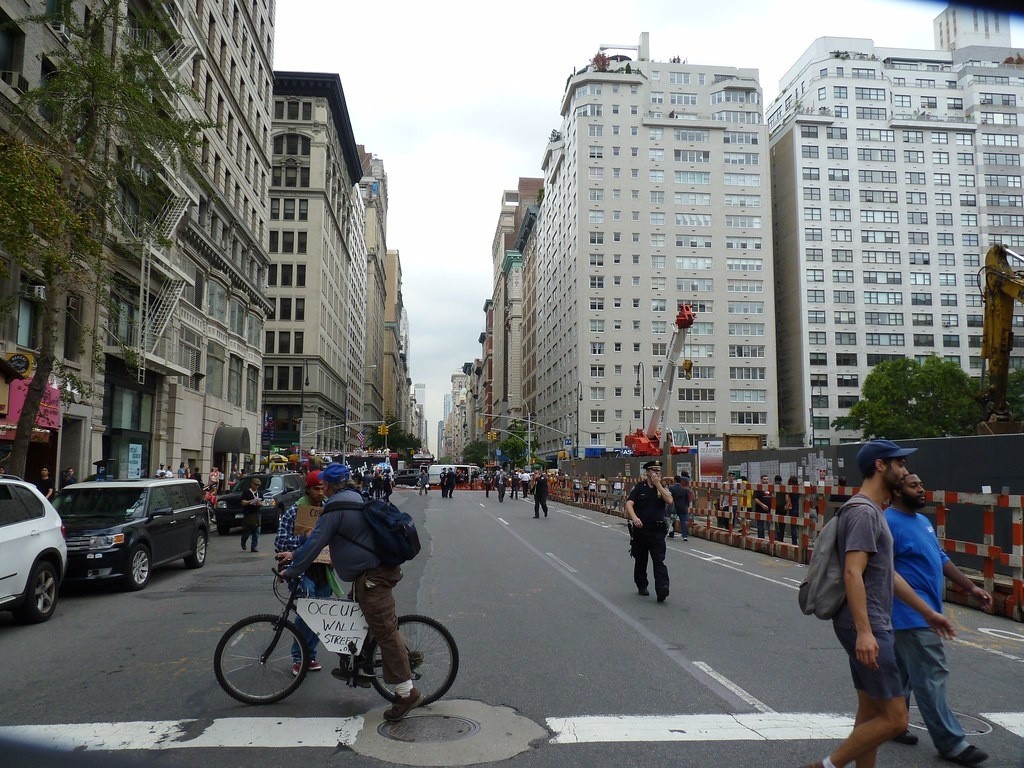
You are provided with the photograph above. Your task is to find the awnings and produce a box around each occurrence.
[215,426,250,454]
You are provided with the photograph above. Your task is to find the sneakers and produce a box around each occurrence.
[292,662,300,674]
[331,667,372,688]
[308,661,322,671]
[381,686,423,721]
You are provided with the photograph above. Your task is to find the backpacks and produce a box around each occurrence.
[320,490,421,565]
[797,498,882,621]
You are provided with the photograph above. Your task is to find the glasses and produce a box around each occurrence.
[255,484,259,486]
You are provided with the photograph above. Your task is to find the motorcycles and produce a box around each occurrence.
[201,482,218,524]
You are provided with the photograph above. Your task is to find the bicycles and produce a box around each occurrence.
[213,548,461,707]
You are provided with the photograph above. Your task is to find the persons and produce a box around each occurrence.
[625,461,673,603]
[275,462,422,721]
[350,468,392,502]
[416,470,428,495]
[530,472,548,518]
[155,462,246,495]
[274,469,334,676]
[241,478,265,551]
[0,466,77,503]
[440,467,465,498]
[883,472,992,766]
[667,475,695,541]
[484,469,531,502]
[829,477,850,516]
[808,439,957,768]
[755,474,800,545]
[715,472,754,534]
[558,468,625,511]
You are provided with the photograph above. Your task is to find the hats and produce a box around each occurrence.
[856,440,917,473]
[252,477,261,484]
[303,469,324,487]
[318,462,349,483]
[643,460,664,471]
[728,470,735,475]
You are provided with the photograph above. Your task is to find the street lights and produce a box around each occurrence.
[384,420,407,450]
[811,382,824,448]
[297,358,311,464]
[576,381,583,458]
[505,392,532,472]
[343,363,378,468]
[635,360,647,433]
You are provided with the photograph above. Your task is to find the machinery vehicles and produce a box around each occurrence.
[973,242,1024,437]
[621,304,700,456]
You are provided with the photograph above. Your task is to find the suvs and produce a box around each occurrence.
[46,477,211,592]
[0,474,68,624]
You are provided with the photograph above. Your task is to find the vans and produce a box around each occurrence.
[215,470,301,534]
[391,469,422,486]
[428,464,482,486]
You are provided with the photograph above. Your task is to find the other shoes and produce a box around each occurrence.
[683,535,688,541]
[893,729,919,744]
[656,586,669,602]
[804,760,824,768]
[534,516,539,518]
[666,536,674,539]
[544,510,548,517]
[251,546,258,552]
[950,744,988,765]
[241,540,246,550]
[638,587,649,595]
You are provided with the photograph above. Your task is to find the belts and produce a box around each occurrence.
[641,522,657,528]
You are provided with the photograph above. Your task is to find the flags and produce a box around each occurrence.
[357,429,365,449]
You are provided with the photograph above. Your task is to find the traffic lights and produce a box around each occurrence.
[493,432,496,440]
[487,432,491,440]
[377,425,383,436]
[384,426,389,436]
